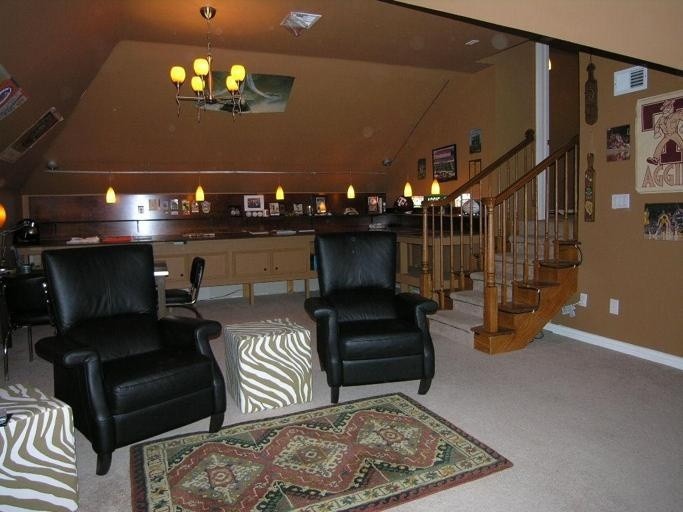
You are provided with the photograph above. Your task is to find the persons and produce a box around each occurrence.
[644,205,682,242]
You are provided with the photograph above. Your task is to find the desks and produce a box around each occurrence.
[0,382,80,512]
[225,318,312,414]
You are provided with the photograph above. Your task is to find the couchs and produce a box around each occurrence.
[30,242,227,476]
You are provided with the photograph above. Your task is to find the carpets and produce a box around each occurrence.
[129,392,513,512]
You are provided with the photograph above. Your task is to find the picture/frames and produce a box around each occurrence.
[244,195,264,212]
[315,197,327,213]
[469,158,481,185]
[432,145,457,181]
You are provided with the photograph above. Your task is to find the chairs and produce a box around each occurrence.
[156,252,209,322]
[304,230,438,403]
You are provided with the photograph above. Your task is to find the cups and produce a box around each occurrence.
[23,265,31,275]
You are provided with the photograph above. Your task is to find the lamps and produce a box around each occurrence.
[275,174,285,200]
[430,178,441,195]
[346,175,356,200]
[0,219,38,267]
[195,174,205,202]
[170,6,250,122]
[106,172,117,205]
[403,181,413,198]
[0,203,7,230]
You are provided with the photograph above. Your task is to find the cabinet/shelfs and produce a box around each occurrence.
[395,235,484,300]
[233,236,316,307]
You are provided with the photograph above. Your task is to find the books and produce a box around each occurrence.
[183,231,215,237]
[132,235,152,240]
[367,195,386,215]
[274,228,296,236]
[64,233,99,244]
[297,227,316,232]
[100,235,132,241]
[247,230,269,235]
